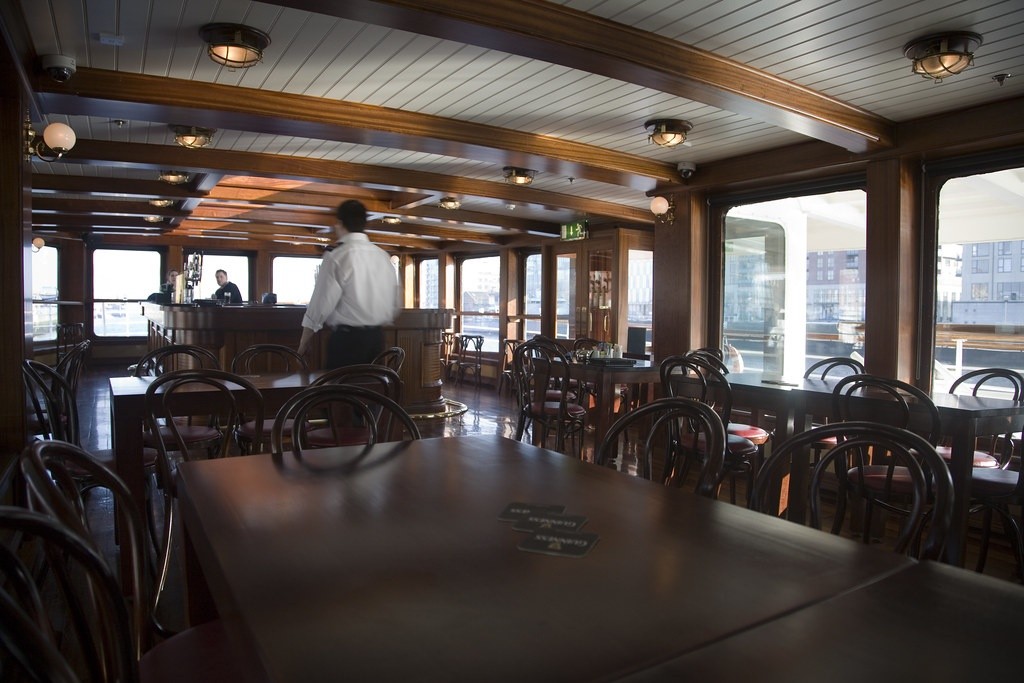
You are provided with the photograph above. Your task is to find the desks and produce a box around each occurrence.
[610,558,1024,682]
[176,435,915,683]
[787,390,1024,570]
[669,372,910,516]
[528,356,673,471]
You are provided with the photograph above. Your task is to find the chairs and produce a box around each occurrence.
[0,323,1024,683]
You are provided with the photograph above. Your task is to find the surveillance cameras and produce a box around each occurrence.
[677,162,696,178]
[41,54,76,83]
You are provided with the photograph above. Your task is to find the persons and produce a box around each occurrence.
[159,270,179,294]
[214,269,242,303]
[295,199,403,373]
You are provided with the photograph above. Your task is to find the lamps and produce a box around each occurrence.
[903,30,983,84]
[651,194,676,225]
[383,216,401,224]
[149,199,173,207]
[199,23,271,70]
[644,120,693,148]
[144,216,163,222]
[439,197,461,209]
[503,166,536,185]
[160,170,190,185]
[35,122,76,162]
[168,123,217,149]
[32,238,44,253]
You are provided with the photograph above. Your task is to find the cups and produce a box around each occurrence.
[224,292,231,304]
[591,351,599,358]
[613,352,622,358]
